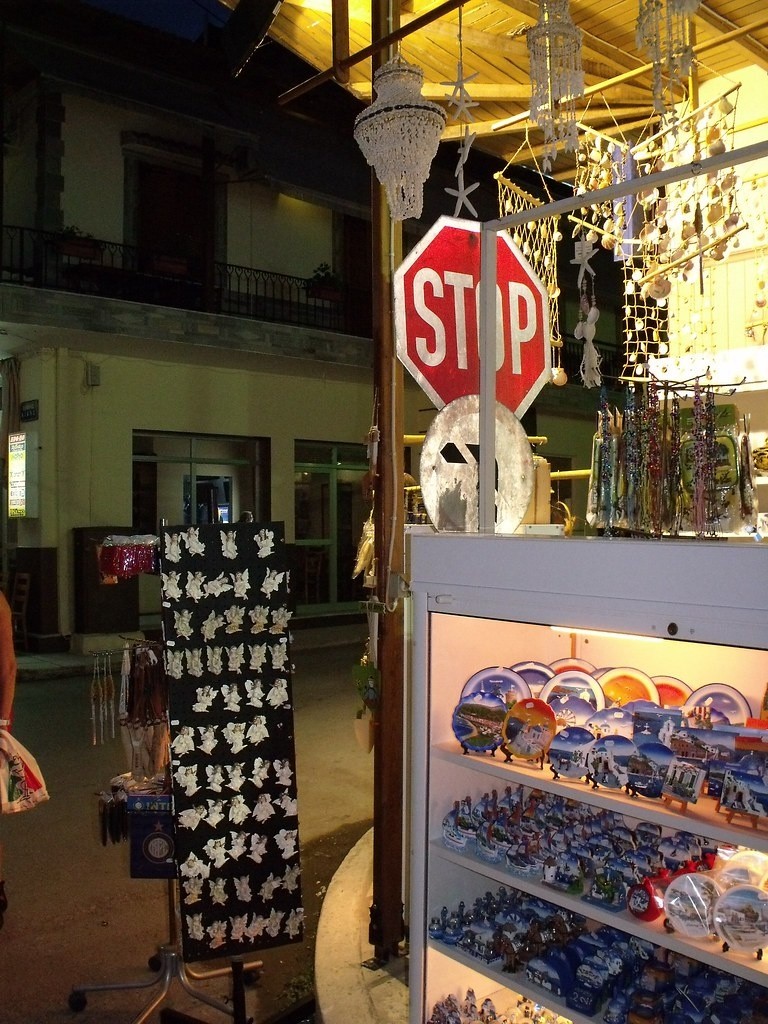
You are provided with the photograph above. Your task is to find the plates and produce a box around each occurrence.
[549,696,597,729]
[715,868,761,894]
[597,667,660,708]
[651,676,693,710]
[549,727,596,778]
[548,658,597,676]
[724,851,768,874]
[509,661,556,698]
[502,699,556,760]
[759,874,768,893]
[627,743,676,798]
[452,692,507,751]
[685,683,752,727]
[621,700,661,714]
[584,708,634,742]
[713,885,768,950]
[588,735,638,788]
[539,671,606,712]
[664,873,723,938]
[591,667,614,680]
[460,666,532,713]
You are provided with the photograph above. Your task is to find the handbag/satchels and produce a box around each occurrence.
[0,729,50,815]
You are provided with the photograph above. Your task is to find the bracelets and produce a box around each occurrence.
[0,719,9,726]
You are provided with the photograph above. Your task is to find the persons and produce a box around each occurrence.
[240,511,254,523]
[0,589,18,924]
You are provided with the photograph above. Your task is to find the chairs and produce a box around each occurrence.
[0,572,32,655]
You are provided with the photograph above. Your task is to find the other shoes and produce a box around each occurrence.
[0,880,8,912]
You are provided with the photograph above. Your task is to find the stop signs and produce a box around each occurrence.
[391,215,555,431]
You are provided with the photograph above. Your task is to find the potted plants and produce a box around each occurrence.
[307,262,347,303]
[54,223,102,260]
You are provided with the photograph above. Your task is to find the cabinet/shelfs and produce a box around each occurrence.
[410,533,768,1024]
